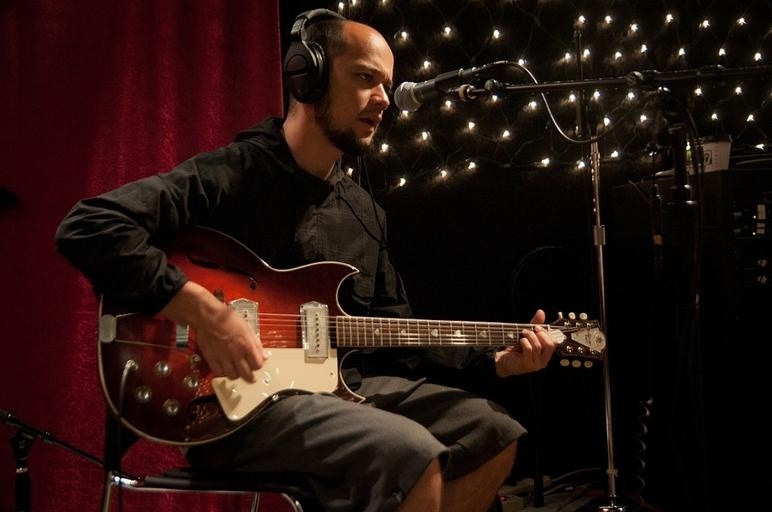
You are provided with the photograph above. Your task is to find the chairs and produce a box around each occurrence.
[102,401,304,511]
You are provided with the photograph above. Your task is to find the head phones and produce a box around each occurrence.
[282,8,347,104]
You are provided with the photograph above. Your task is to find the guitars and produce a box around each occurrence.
[95,223,608,448]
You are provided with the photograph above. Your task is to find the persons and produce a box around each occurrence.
[54,8,558,512]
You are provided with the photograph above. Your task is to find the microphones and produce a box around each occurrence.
[394,66,477,112]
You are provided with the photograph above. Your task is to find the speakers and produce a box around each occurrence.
[609,168,772,512]
